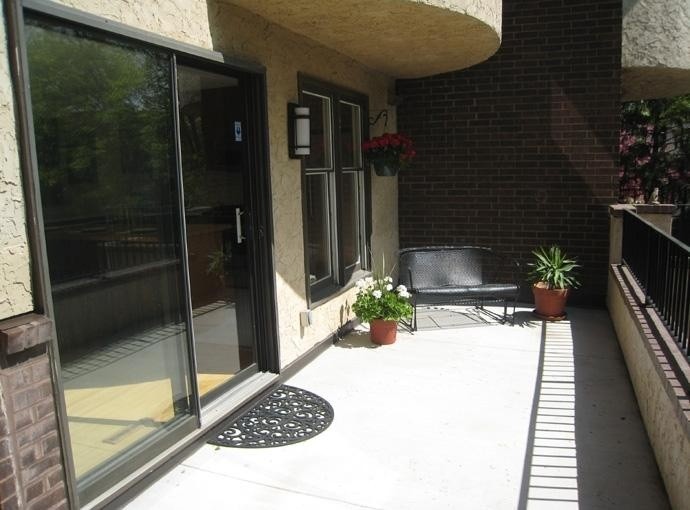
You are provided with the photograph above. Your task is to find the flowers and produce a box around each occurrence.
[351,243,414,324]
[362,132,416,162]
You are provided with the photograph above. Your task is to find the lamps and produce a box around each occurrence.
[287,101,312,160]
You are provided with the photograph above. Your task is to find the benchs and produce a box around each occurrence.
[396,245,521,333]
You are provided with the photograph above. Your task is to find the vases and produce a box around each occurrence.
[368,317,398,345]
[372,156,402,176]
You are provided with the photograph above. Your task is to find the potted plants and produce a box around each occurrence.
[524,244,586,317]
